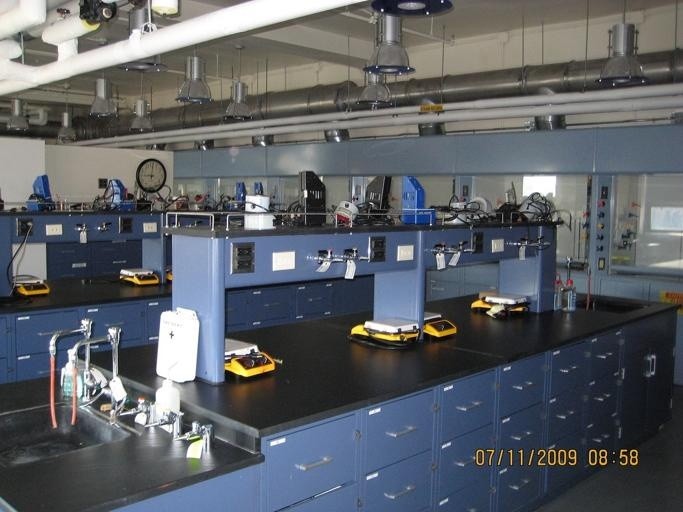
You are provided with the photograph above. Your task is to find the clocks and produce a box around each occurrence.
[137,159,168,193]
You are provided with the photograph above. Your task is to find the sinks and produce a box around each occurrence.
[1,397,130,473]
[576,297,649,314]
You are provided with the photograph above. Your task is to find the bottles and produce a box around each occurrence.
[154,379,181,434]
[560,280,577,312]
[553,278,562,310]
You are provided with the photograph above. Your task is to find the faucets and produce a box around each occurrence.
[565,255,572,280]
[67,326,123,425]
[49,316,94,401]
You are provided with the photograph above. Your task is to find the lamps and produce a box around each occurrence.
[596,3,647,85]
[59,69,152,148]
[359,12,415,109]
[5,97,29,131]
[176,45,252,122]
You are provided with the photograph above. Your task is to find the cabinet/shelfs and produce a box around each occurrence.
[47,241,143,278]
[618,311,678,450]
[599,276,682,385]
[548,329,618,504]
[0,298,173,381]
[437,370,497,512]
[362,388,438,510]
[425,265,499,300]
[262,409,361,510]
[227,277,373,333]
[498,352,548,511]
